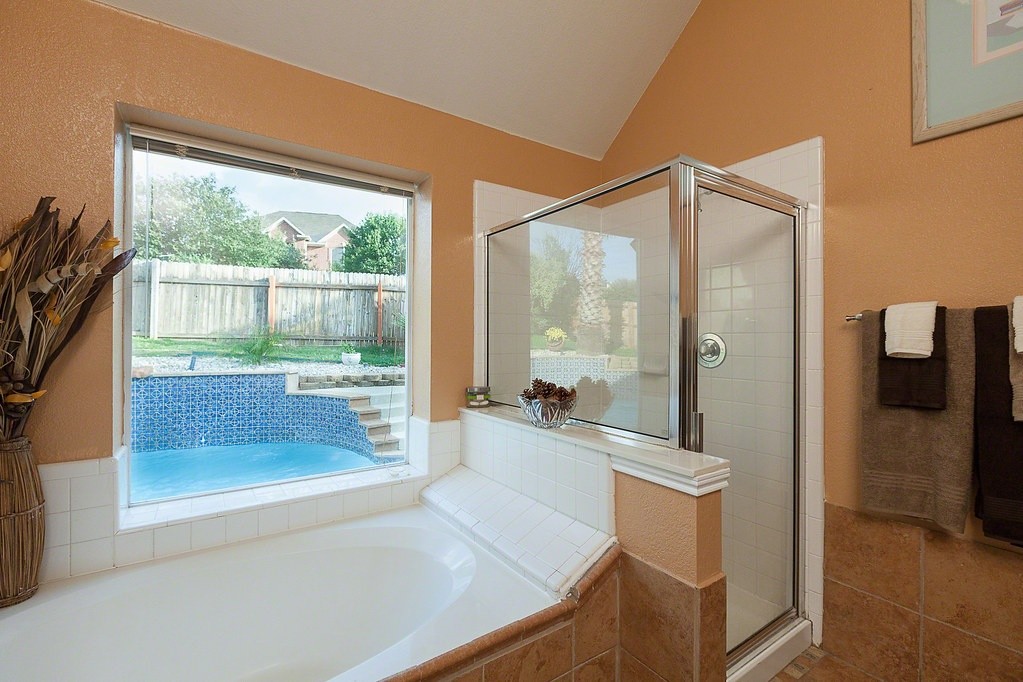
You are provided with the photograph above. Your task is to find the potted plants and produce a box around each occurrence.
[340,343,361,365]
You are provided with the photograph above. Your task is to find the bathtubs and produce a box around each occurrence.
[6,503,571,682]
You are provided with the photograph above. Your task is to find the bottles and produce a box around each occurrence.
[466,386,490,408]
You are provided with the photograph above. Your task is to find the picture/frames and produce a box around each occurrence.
[911,0,1023,144]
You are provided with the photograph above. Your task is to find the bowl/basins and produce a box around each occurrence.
[517,395,579,429]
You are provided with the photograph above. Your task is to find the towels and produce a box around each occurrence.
[853,294,1023,545]
